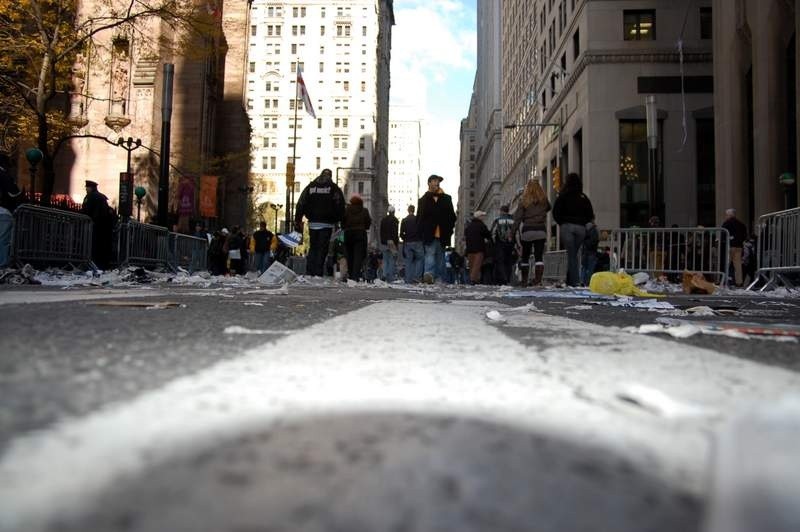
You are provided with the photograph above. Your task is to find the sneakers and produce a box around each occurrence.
[424,271,434,285]
[470,280,479,285]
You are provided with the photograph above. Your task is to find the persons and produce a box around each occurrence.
[552,173,594,287]
[621,216,710,281]
[341,193,372,282]
[416,172,456,283]
[490,205,515,285]
[465,210,490,284]
[192,219,288,277]
[449,246,461,283]
[380,206,400,284]
[400,204,424,285]
[511,178,551,289]
[1,149,23,279]
[80,180,114,270]
[720,208,747,287]
[326,227,383,281]
[581,217,598,286]
[295,168,345,276]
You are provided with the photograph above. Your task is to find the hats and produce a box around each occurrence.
[694,225,706,233]
[85,179,98,188]
[649,216,658,225]
[474,210,486,219]
[427,175,443,184]
[221,228,228,233]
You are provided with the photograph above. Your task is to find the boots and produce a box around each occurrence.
[533,262,544,288]
[517,264,529,289]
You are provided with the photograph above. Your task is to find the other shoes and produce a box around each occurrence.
[713,280,722,287]
[735,283,745,289]
[499,279,508,284]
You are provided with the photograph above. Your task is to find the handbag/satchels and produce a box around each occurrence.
[277,224,304,249]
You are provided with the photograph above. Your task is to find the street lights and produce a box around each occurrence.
[135,186,147,266]
[119,137,141,264]
[504,120,563,251]
[27,148,43,270]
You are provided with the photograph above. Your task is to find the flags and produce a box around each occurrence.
[177,174,194,214]
[199,175,218,217]
[296,65,316,119]
